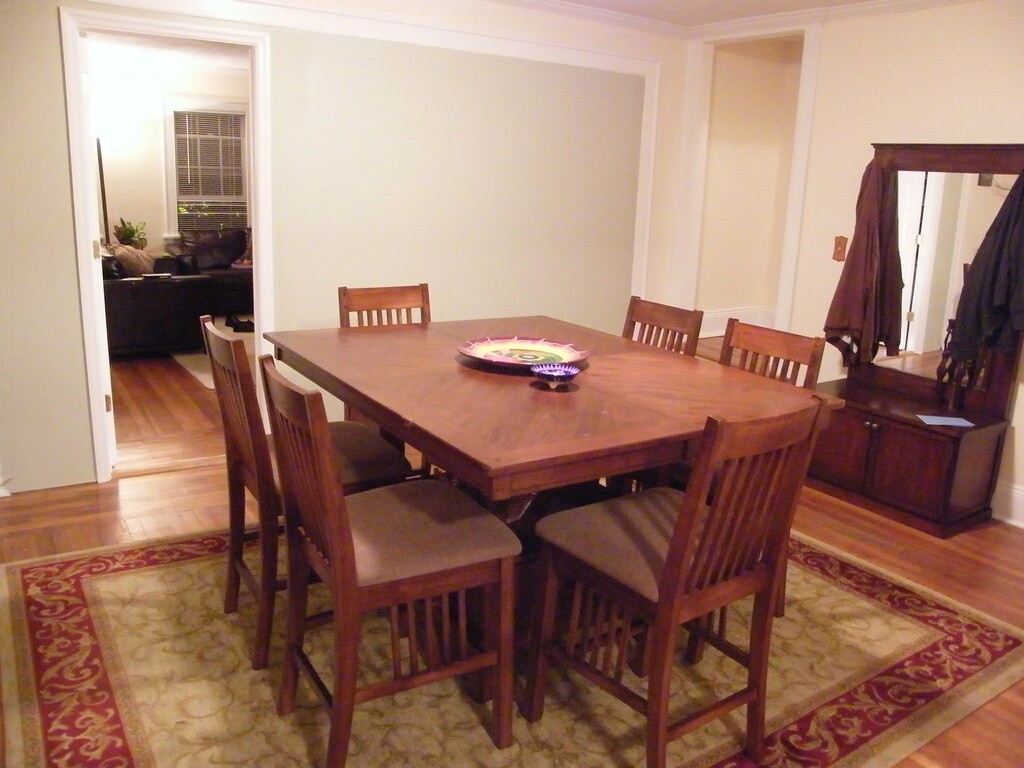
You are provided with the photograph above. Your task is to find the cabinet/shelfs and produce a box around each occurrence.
[796,379,1009,540]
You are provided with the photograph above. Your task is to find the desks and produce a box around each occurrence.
[263,316,846,723]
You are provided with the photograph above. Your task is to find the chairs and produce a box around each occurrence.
[590,295,704,494]
[259,353,522,768]
[339,283,445,482]
[523,394,829,768]
[199,313,415,670]
[632,318,826,617]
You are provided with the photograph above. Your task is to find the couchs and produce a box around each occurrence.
[99,228,254,356]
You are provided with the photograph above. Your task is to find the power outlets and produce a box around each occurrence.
[832,235,847,262]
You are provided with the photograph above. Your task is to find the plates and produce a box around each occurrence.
[457,336,590,369]
[531,363,581,386]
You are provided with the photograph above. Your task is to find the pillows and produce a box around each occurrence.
[112,244,155,276]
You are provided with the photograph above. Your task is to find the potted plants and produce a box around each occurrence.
[112,217,147,250]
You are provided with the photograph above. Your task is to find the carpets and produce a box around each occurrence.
[0,517,1024,768]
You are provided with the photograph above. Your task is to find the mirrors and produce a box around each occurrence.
[867,171,1019,392]
